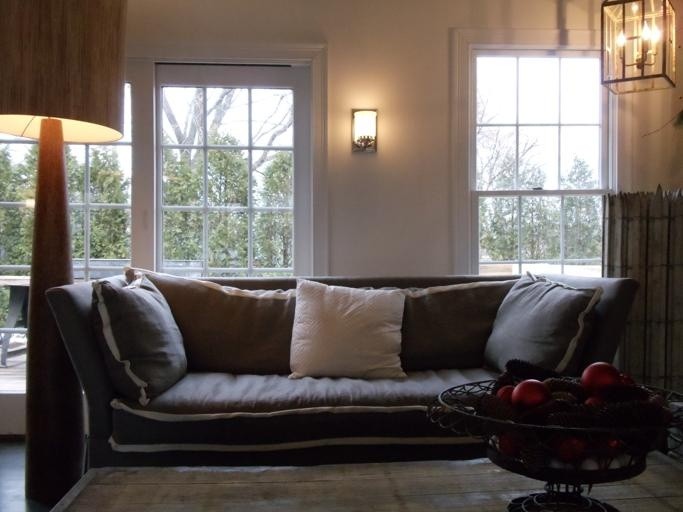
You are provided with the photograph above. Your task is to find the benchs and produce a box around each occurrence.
[0,328,30,368]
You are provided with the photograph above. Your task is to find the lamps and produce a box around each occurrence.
[600,0,675,94]
[0,1,128,505]
[351,109,377,152]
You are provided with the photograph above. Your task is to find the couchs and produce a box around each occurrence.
[43,274,641,479]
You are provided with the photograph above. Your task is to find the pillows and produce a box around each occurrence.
[93,269,188,408]
[484,270,604,373]
[287,278,409,380]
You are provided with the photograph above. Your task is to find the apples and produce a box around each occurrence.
[488,362,636,459]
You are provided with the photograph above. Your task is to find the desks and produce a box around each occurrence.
[0,276,30,368]
